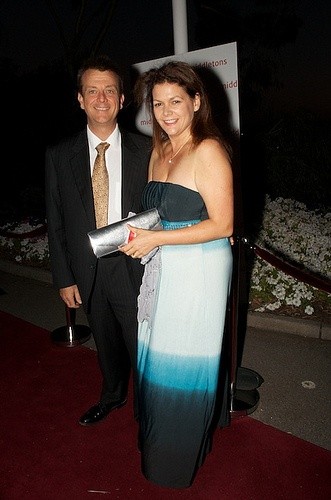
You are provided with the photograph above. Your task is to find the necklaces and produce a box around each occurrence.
[168,135,191,163]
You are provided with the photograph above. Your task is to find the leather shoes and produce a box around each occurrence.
[80,395,127,426]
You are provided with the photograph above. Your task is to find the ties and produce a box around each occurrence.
[92,142,110,229]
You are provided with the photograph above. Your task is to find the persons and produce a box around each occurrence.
[117,60,235,489]
[43,54,234,427]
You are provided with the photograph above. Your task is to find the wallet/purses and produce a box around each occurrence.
[88,208,163,258]
